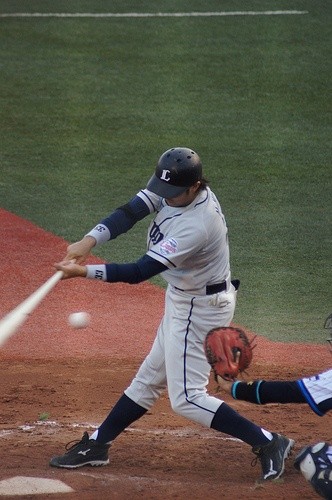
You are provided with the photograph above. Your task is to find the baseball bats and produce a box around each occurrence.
[0,259,76,346]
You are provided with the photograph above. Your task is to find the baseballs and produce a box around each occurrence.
[68,312,88,329]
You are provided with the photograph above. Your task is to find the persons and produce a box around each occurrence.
[205,312,331,499]
[50,148,295,483]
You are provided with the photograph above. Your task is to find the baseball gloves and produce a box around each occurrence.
[204,327,257,396]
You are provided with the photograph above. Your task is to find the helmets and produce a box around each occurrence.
[147,147,202,199]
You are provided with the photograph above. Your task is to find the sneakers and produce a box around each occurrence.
[50,431,111,469]
[252,432,295,482]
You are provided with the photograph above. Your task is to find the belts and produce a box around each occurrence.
[175,279,240,295]
